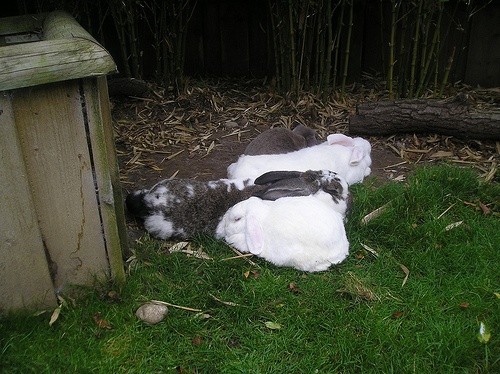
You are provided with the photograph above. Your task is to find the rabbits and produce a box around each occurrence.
[127,124,372,273]
